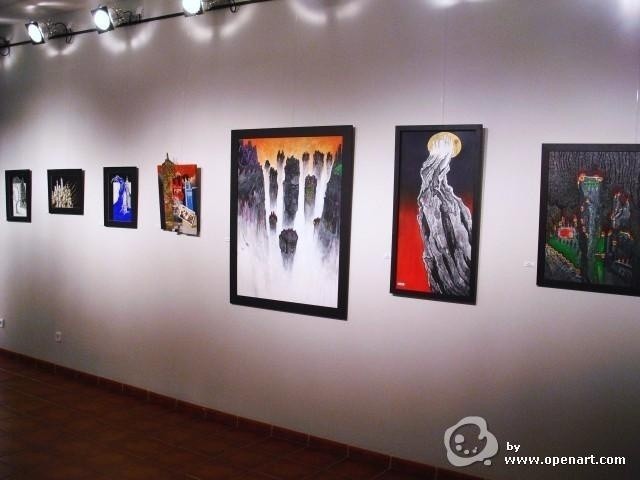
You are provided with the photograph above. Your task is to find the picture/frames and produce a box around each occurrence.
[230,123,355,321]
[103,166,138,228]
[47,168,84,215]
[390,123,484,305]
[4,168,31,223]
[536,143,640,297]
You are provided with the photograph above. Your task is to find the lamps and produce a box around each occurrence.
[183,0,215,17]
[25,21,56,46]
[91,5,132,34]
